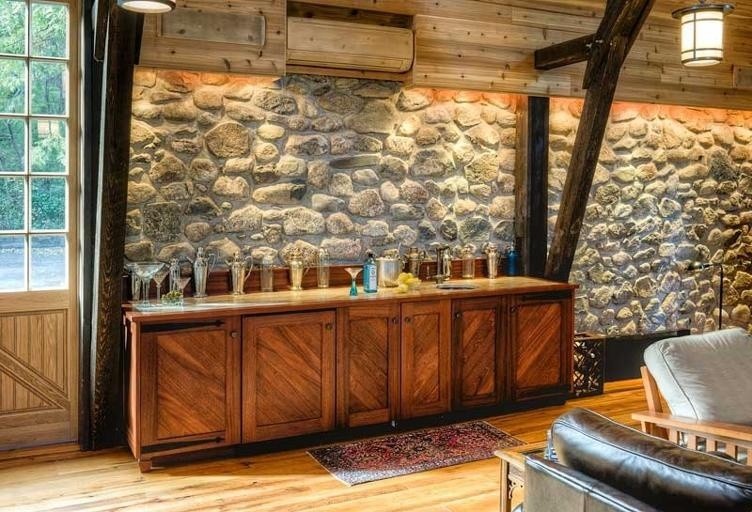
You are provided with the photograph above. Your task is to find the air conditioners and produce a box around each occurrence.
[286,17,414,73]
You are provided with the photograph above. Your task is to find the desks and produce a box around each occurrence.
[494,440,548,512]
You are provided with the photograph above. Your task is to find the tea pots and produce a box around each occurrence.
[286,248,310,291]
[403,247,426,279]
[224,251,254,296]
[484,245,501,279]
[187,247,217,299]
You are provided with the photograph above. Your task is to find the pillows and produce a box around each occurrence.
[643,327,752,443]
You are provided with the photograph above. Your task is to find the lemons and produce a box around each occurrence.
[396,272,420,293]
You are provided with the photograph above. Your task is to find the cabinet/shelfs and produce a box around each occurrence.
[505,286,566,413]
[241,304,336,454]
[344,295,446,437]
[119,316,234,461]
[452,290,505,417]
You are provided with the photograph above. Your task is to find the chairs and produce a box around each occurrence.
[631,365,752,467]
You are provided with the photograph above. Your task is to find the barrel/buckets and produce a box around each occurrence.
[375,258,405,288]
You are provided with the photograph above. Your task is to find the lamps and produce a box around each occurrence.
[117,0,175,14]
[671,1,734,68]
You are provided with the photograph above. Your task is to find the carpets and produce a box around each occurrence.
[306,419,529,486]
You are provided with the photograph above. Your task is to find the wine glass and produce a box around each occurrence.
[127,261,170,308]
[175,278,191,306]
[345,267,364,296]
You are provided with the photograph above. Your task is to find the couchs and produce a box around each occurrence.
[523,407,752,512]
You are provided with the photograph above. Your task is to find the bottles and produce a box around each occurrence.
[260,255,274,291]
[362,252,378,293]
[505,241,519,276]
[169,258,181,292]
[436,245,453,281]
[462,246,475,280]
[316,246,330,288]
